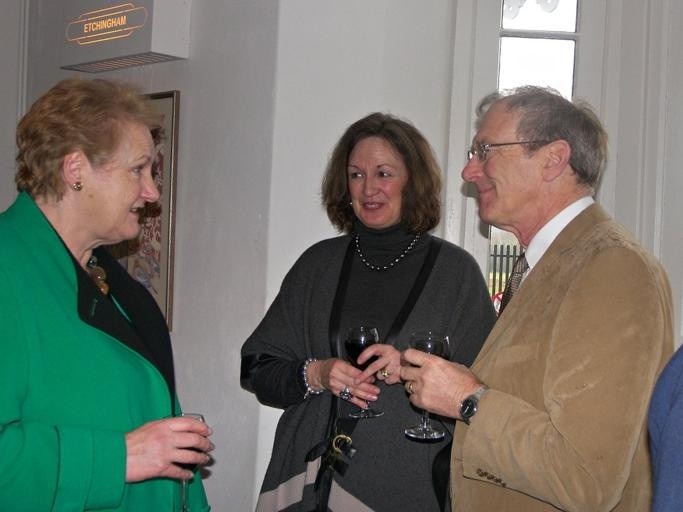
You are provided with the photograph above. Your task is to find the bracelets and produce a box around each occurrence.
[301,357,325,400]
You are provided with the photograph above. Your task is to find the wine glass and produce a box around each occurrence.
[336,325,386,419]
[163,413,206,512]
[403,329,451,441]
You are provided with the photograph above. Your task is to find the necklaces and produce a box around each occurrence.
[86,252,110,296]
[355,229,422,271]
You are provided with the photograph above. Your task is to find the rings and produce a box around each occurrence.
[379,366,392,378]
[338,384,354,400]
[407,379,416,395]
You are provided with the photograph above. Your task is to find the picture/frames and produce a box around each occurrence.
[104,90,180,333]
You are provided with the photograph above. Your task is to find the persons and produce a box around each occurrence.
[0,78,216,512]
[237,110,498,510]
[399,83,674,512]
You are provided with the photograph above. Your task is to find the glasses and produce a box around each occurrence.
[467,140,550,161]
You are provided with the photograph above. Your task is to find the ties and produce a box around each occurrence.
[498,252,529,319]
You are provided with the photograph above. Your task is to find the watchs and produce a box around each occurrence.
[458,382,491,426]
